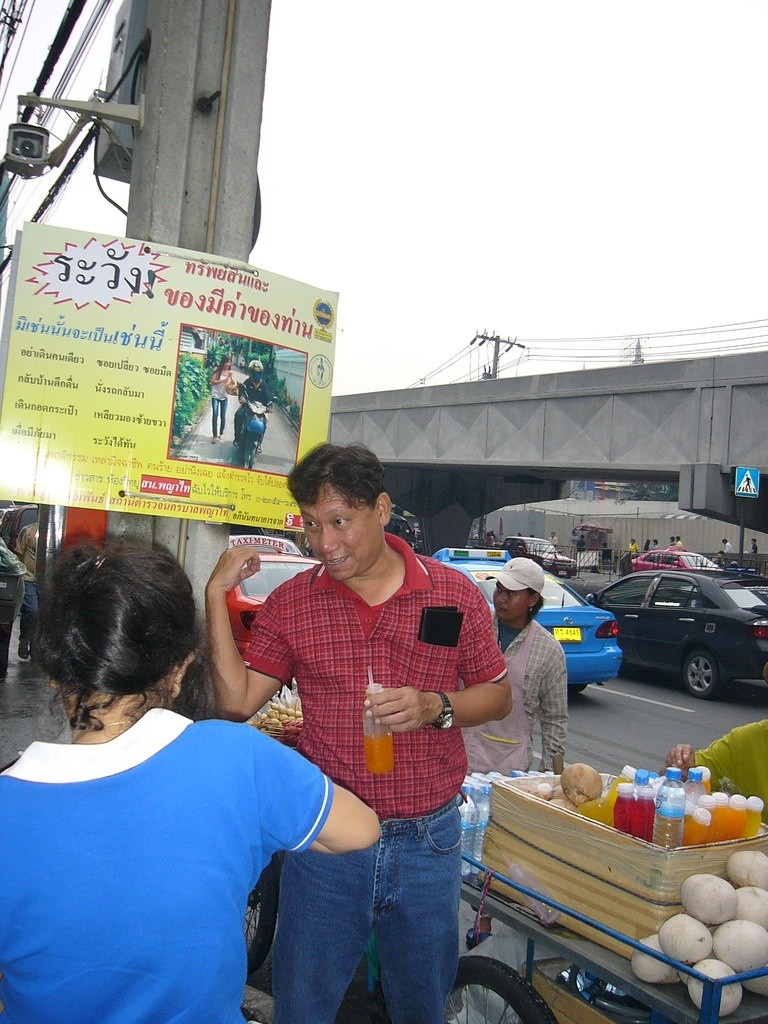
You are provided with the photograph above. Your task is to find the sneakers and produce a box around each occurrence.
[18,642,29,659]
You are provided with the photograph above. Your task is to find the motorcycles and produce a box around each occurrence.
[236,382,279,469]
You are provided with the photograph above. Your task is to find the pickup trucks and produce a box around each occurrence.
[489,536,578,578]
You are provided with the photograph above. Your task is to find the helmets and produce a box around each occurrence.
[248,360,264,373]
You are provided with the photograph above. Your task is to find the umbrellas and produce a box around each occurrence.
[575,519,613,534]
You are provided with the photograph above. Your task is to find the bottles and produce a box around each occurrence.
[501,854,562,925]
[461,771,556,876]
[362,683,394,773]
[596,766,761,848]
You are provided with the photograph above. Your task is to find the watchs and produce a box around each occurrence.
[431,692,455,730]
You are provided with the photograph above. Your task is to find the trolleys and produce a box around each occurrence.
[245,847,768,1024]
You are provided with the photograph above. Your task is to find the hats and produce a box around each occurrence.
[485,556,545,594]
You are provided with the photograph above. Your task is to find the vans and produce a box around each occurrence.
[384,513,414,544]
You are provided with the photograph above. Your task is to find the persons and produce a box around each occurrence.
[0,536,26,682]
[459,557,569,950]
[203,443,513,1024]
[13,522,40,663]
[750,538,758,553]
[662,661,768,827]
[484,530,558,550]
[602,543,612,568]
[0,534,384,1024]
[722,538,733,554]
[628,536,683,559]
[210,355,273,454]
[717,551,725,565]
[576,534,586,553]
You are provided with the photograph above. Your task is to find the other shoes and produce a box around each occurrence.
[233,438,239,445]
[219,435,224,440]
[257,445,262,453]
[211,437,219,444]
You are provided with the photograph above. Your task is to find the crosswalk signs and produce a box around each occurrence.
[734,466,760,498]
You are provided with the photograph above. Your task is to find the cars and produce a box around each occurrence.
[0,501,39,544]
[440,561,623,692]
[631,550,723,574]
[584,569,768,699]
[223,551,323,657]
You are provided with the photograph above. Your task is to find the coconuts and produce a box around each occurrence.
[630,848,768,1016]
[545,762,603,812]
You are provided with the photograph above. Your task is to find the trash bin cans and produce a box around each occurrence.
[602,548,614,570]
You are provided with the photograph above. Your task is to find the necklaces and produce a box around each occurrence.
[102,721,134,727]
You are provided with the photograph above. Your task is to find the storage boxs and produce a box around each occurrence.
[478,773,768,960]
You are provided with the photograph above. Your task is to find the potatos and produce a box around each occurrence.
[256,703,303,724]
